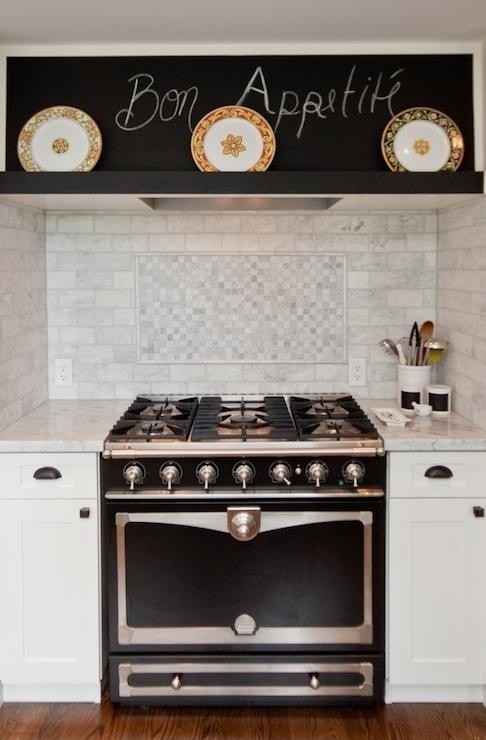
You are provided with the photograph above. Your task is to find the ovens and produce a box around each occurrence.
[99,452,387,710]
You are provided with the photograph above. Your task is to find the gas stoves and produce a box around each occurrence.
[104,393,385,443]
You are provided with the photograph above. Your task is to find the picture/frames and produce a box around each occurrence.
[132,251,348,366]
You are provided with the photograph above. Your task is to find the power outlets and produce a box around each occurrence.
[55,357,72,388]
[348,357,367,388]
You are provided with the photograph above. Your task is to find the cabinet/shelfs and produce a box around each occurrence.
[1,451,104,706]
[384,451,485,707]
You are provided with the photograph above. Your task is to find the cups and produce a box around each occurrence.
[400,384,452,418]
[398,364,431,406]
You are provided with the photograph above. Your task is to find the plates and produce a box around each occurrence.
[372,407,412,427]
[381,107,466,173]
[190,105,277,171]
[16,106,104,172]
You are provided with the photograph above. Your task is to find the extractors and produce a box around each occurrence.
[138,197,345,211]
